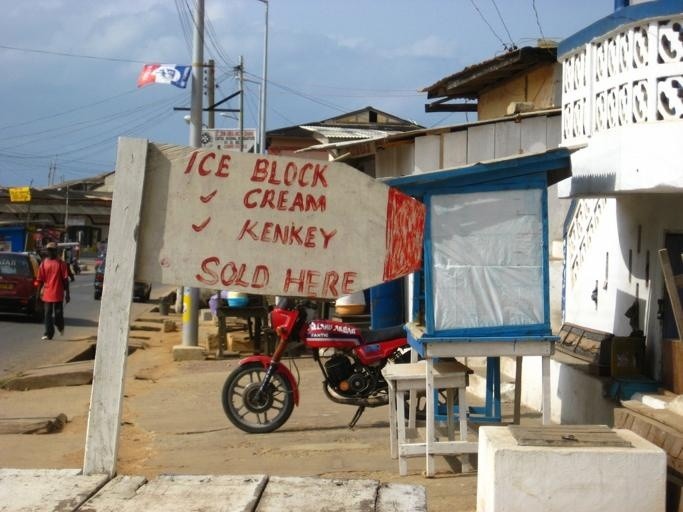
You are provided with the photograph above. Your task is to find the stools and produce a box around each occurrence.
[386,358,472,477]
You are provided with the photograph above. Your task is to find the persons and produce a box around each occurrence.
[66,247,75,281]
[33,248,70,340]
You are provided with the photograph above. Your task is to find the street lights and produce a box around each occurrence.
[183,114,215,131]
[56,187,68,242]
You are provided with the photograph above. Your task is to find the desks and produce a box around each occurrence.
[403,320,559,474]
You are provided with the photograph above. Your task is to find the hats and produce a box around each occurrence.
[46,242,57,249]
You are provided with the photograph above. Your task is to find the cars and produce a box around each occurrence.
[90,251,152,303]
[0,249,45,322]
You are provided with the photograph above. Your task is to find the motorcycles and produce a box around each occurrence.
[221,294,471,435]
